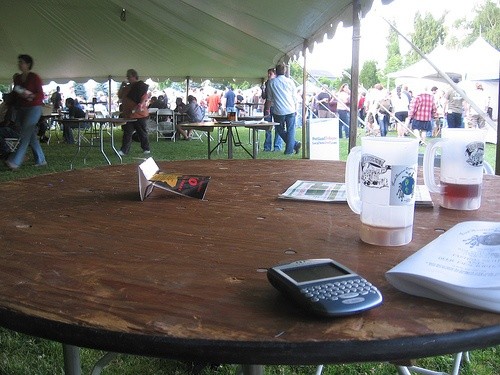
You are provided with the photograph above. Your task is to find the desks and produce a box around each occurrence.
[41,114,64,145]
[208,115,270,155]
[53,118,137,170]
[0,159,500,375]
[179,120,280,159]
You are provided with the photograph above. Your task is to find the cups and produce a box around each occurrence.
[345,135,419,248]
[423,127,485,210]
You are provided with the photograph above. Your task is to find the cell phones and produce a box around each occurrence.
[267,257,383,318]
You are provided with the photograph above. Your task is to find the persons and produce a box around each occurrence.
[116,69,150,156]
[262,67,282,152]
[467,82,489,129]
[296,79,446,137]
[406,90,440,146]
[61,98,87,145]
[442,75,467,128]
[49,86,62,131]
[147,95,205,141]
[188,86,261,123]
[265,62,301,155]
[0,54,49,171]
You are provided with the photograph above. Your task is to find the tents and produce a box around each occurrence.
[385,37,500,145]
[0,0,374,160]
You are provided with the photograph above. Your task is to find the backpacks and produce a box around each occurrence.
[379,99,390,114]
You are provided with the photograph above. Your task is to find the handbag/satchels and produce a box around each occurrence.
[37,121,50,137]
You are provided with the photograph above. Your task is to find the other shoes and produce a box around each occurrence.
[295,142,301,154]
[4,160,18,169]
[31,162,47,166]
[118,151,125,155]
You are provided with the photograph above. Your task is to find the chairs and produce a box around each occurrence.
[95,111,111,136]
[156,109,176,143]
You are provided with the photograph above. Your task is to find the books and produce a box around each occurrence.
[414,185,435,208]
[385,221,500,314]
